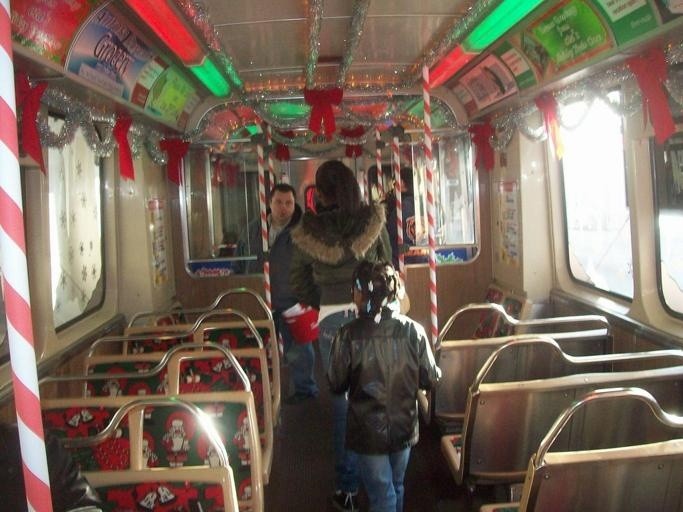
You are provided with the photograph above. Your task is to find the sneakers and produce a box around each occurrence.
[288,392,320,404]
[332,490,360,512]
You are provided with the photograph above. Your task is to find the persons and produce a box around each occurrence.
[229,183,319,404]
[0,421,103,511]
[288,160,393,510]
[326,259,444,511]
[385,166,414,269]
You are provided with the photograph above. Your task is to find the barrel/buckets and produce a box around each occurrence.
[283,304,319,343]
